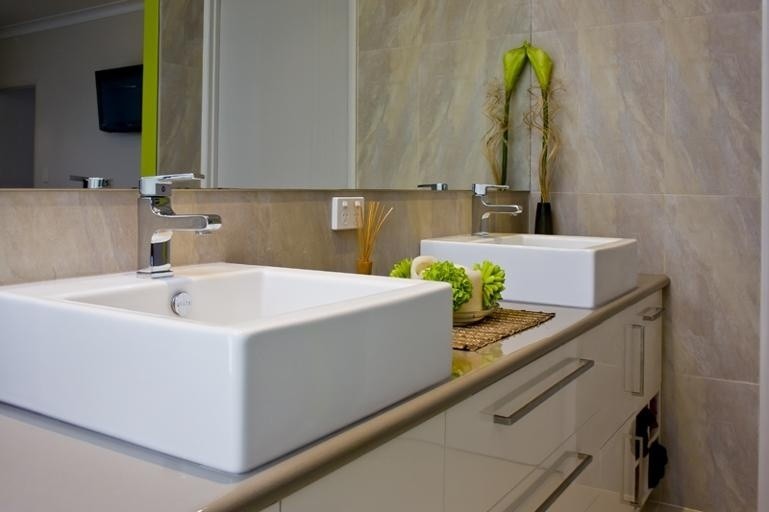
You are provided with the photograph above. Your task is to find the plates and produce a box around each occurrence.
[453,302,499,326]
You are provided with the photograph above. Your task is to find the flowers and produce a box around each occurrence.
[522,40,568,203]
[484,45,527,190]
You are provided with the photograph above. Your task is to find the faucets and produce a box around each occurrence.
[70,174,111,188]
[470,184,522,231]
[136,171,221,278]
[418,182,446,189]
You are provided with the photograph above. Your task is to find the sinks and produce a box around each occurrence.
[419,232,637,307]
[53,260,454,475]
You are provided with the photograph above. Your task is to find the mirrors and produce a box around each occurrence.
[0,0,531,192]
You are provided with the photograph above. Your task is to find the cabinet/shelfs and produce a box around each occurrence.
[445,290,663,512]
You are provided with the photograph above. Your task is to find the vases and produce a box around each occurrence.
[535,201,554,234]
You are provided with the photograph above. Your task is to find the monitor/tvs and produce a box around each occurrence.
[94,64,142,133]
